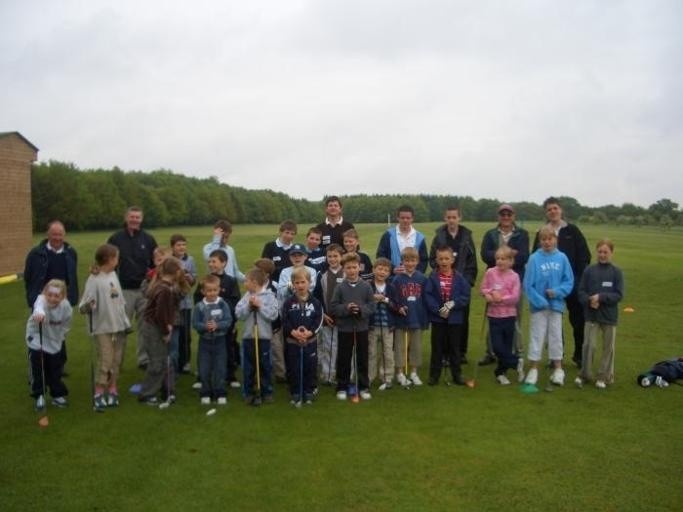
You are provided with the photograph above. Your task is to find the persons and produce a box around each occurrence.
[25,279,73,412]
[328,252,375,401]
[24,220,79,377]
[141,245,193,400]
[137,256,184,405]
[318,195,360,251]
[193,249,241,389]
[106,205,157,319]
[575,239,625,389]
[279,243,316,304]
[428,207,479,289]
[191,275,231,405]
[320,243,346,387]
[303,226,328,278]
[532,197,592,368]
[376,205,425,273]
[341,230,371,273]
[480,203,529,371]
[261,219,298,292]
[394,247,427,387]
[170,234,193,375]
[78,244,132,407]
[367,257,396,390]
[281,266,325,408]
[424,244,469,387]
[480,246,524,385]
[522,226,574,386]
[234,269,279,406]
[253,259,285,384]
[202,220,246,285]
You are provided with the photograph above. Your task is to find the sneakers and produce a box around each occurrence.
[360,390,372,401]
[244,396,254,405]
[51,397,70,409]
[516,357,526,383]
[304,394,313,405]
[594,380,607,389]
[410,372,424,386]
[496,375,510,386]
[264,396,274,404]
[107,391,120,407]
[383,376,393,389]
[201,396,211,404]
[93,393,107,408]
[289,395,300,406]
[191,382,203,390]
[397,373,411,387]
[549,368,565,386]
[35,396,46,409]
[478,355,494,366]
[217,397,227,406]
[336,391,347,401]
[575,376,585,385]
[142,396,158,407]
[524,369,539,385]
[230,381,241,389]
[453,375,466,385]
[427,377,439,386]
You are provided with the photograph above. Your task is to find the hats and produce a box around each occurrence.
[288,244,308,257]
[497,204,515,213]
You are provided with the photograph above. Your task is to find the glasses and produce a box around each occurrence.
[500,212,514,216]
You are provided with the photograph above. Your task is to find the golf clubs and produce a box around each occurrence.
[404,306,412,390]
[377,302,387,392]
[350,314,359,403]
[159,342,171,409]
[205,331,217,415]
[251,307,263,406]
[465,302,489,388]
[543,298,553,392]
[295,328,305,408]
[444,318,452,387]
[88,310,105,413]
[324,327,334,387]
[574,308,594,389]
[38,321,49,426]
